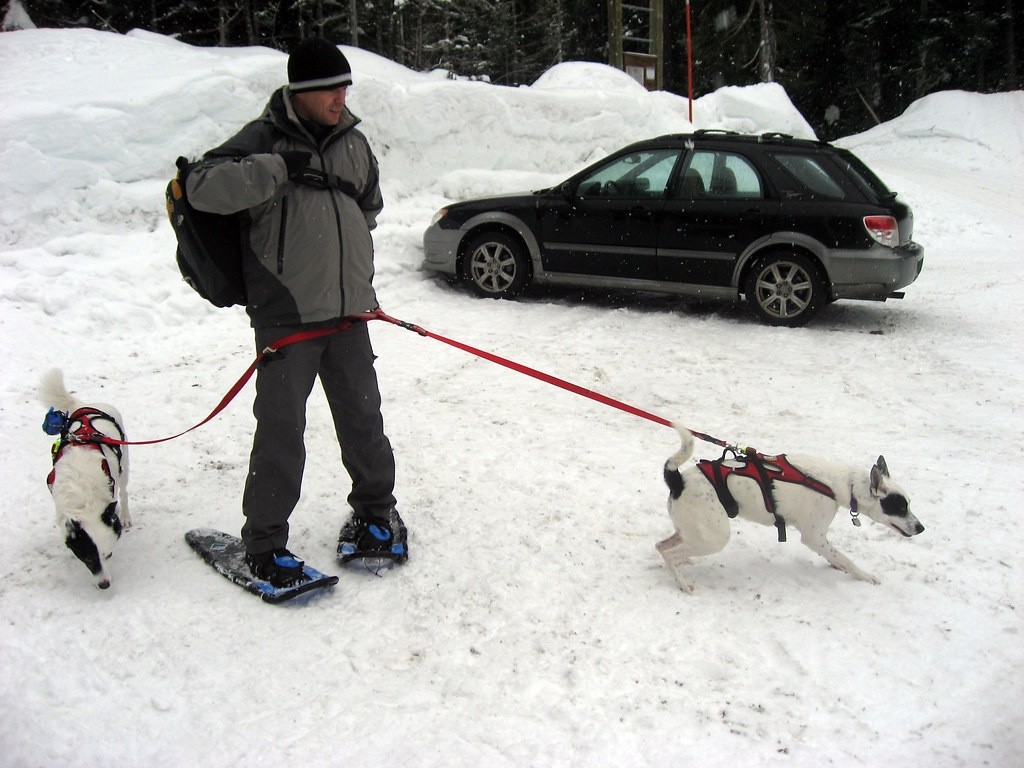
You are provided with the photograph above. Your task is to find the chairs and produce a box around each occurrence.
[707,167,740,198]
[676,168,707,198]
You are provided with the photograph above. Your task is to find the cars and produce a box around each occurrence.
[418,127,926,330]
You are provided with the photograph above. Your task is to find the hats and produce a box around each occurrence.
[288,38,352,92]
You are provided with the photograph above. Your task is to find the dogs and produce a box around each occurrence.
[31,365,134,591]
[653,421,926,597]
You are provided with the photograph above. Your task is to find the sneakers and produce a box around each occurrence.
[245,547,305,583]
[354,516,395,557]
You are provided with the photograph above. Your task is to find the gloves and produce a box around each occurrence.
[279,150,312,183]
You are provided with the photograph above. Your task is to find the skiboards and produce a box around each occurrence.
[182,499,411,609]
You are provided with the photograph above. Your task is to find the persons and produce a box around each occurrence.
[187,39,401,584]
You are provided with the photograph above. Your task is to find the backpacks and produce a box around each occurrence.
[165,125,379,307]
